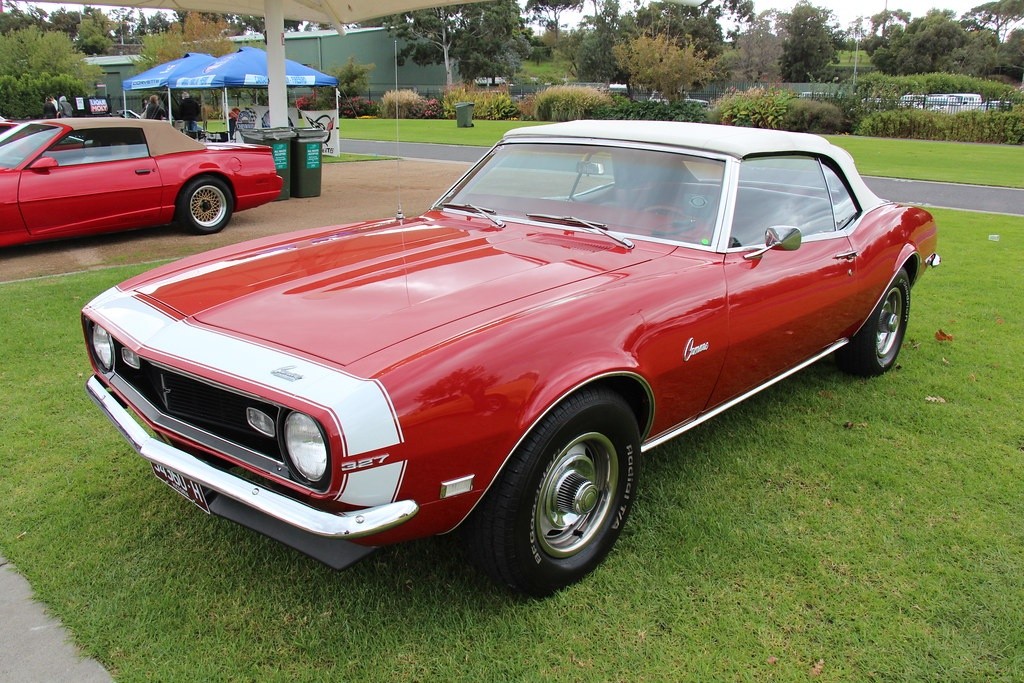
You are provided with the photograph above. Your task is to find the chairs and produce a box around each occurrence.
[81,140,101,162]
[180,128,199,141]
[107,143,128,159]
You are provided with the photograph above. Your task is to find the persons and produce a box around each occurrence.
[106,94,112,115]
[42,96,58,118]
[147,96,165,120]
[177,92,200,141]
[50,96,57,111]
[59,96,73,118]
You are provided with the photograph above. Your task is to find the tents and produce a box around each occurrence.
[169,47,340,156]
[122,53,259,130]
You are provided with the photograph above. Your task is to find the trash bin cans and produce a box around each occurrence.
[240,124,328,203]
[453,98,477,129]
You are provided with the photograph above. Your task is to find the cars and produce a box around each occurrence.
[901,93,1011,115]
[0,121,82,163]
[79,120,939,601]
[0,118,285,248]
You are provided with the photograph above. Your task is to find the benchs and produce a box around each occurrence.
[631,180,829,251]
